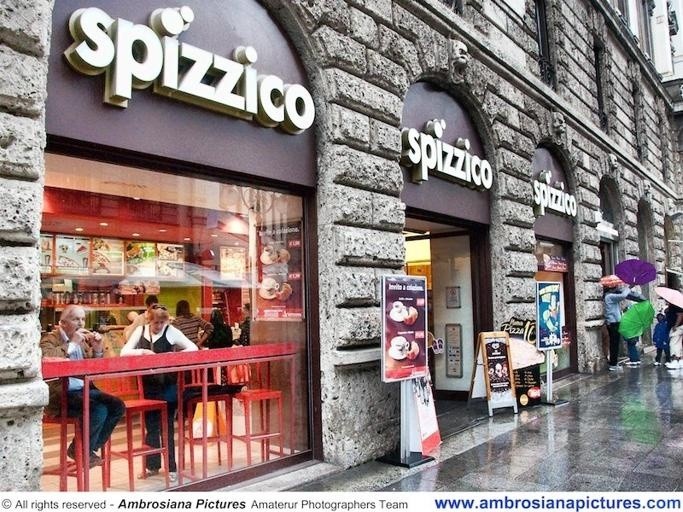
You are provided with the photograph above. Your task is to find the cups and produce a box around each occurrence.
[262,278,280,295]
[390,335,410,354]
[393,300,406,314]
[264,246,274,258]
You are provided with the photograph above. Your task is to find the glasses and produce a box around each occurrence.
[151,305,167,310]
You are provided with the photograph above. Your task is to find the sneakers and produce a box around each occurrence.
[67,443,102,467]
[608,361,640,371]
[653,360,683,369]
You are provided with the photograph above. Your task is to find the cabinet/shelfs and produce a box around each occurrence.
[41,275,252,339]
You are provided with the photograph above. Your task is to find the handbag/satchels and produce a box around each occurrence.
[151,373,177,386]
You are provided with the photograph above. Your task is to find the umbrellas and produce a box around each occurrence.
[616,258,656,284]
[616,300,655,339]
[655,286,683,309]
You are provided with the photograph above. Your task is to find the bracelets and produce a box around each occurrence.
[674,324,678,327]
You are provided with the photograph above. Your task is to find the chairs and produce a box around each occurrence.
[41,360,284,491]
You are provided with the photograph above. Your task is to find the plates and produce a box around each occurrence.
[259,287,277,300]
[388,347,410,360]
[389,308,409,322]
[260,252,278,265]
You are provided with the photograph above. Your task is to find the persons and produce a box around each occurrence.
[601,274,639,370]
[122,295,170,341]
[171,300,214,348]
[233,303,250,346]
[663,303,682,370]
[623,305,642,367]
[652,314,666,366]
[119,305,197,480]
[38,305,125,464]
[206,309,232,347]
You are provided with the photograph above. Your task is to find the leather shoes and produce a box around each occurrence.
[138,469,158,479]
[169,471,177,482]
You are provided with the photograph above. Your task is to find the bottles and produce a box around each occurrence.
[52,290,111,305]
